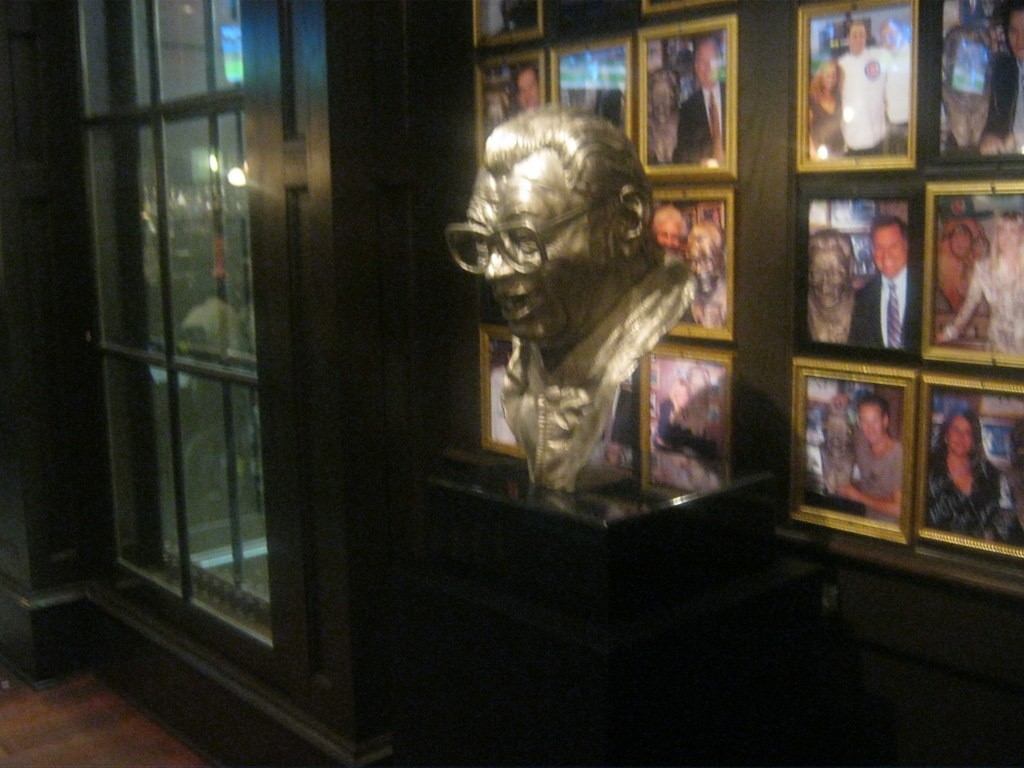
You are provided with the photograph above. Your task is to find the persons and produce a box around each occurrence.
[647,69,686,163]
[445,105,700,492]
[478,0,1024,557]
[816,406,859,497]
[944,22,989,157]
[679,366,714,437]
[179,268,255,484]
[481,63,512,142]
[999,420,1024,533]
[682,220,732,328]
[807,226,860,345]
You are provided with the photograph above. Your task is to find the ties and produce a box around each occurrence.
[888,285,903,350]
[708,92,724,160]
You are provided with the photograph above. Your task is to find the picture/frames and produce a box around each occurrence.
[916,0,1024,182]
[640,0,738,17]
[636,12,738,181]
[472,0,543,48]
[796,0,919,176]
[920,179,1023,370]
[473,47,546,171]
[651,188,736,341]
[639,344,734,500]
[788,356,921,548]
[479,322,525,458]
[914,369,1024,565]
[549,37,633,148]
[798,185,921,367]
